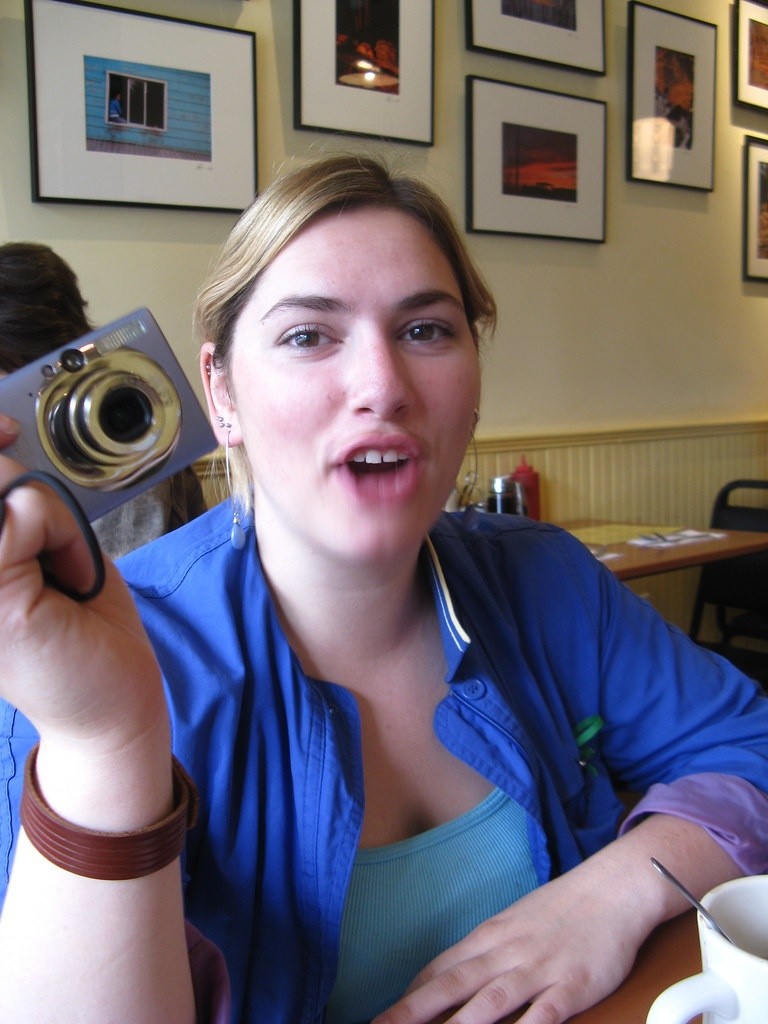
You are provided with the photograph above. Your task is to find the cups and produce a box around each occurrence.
[646,875,767,1024]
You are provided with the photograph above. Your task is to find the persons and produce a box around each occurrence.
[656,49,694,150]
[351,38,399,74]
[109,93,127,123]
[0,242,209,561]
[0,155,768,1022]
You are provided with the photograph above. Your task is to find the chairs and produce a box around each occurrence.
[689,480,768,655]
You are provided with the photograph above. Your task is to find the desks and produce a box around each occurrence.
[552,517,767,583]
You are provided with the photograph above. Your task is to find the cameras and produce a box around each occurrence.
[0,305,218,531]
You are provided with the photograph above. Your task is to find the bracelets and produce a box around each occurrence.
[20,741,200,881]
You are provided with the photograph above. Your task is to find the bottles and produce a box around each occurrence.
[511,454,541,523]
[487,472,516,516]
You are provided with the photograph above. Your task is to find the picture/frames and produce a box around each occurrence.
[23,0,259,213]
[465,75,607,243]
[743,135,768,283]
[734,0,768,114]
[464,0,607,77]
[627,0,718,193]
[293,0,435,146]
